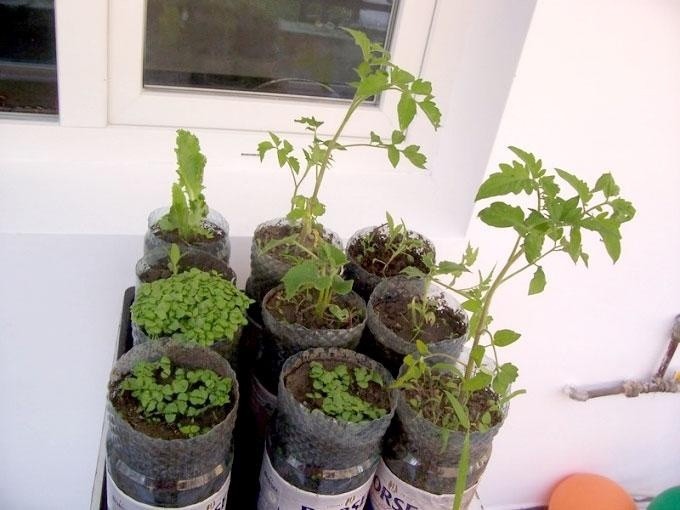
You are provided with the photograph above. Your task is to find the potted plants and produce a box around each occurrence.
[130,267,259,359]
[105,334,239,509]
[362,249,485,377]
[243,25,443,332]
[144,128,232,260]
[259,348,400,509]
[346,214,438,299]
[251,246,370,418]
[366,145,637,509]
[133,243,236,293]
